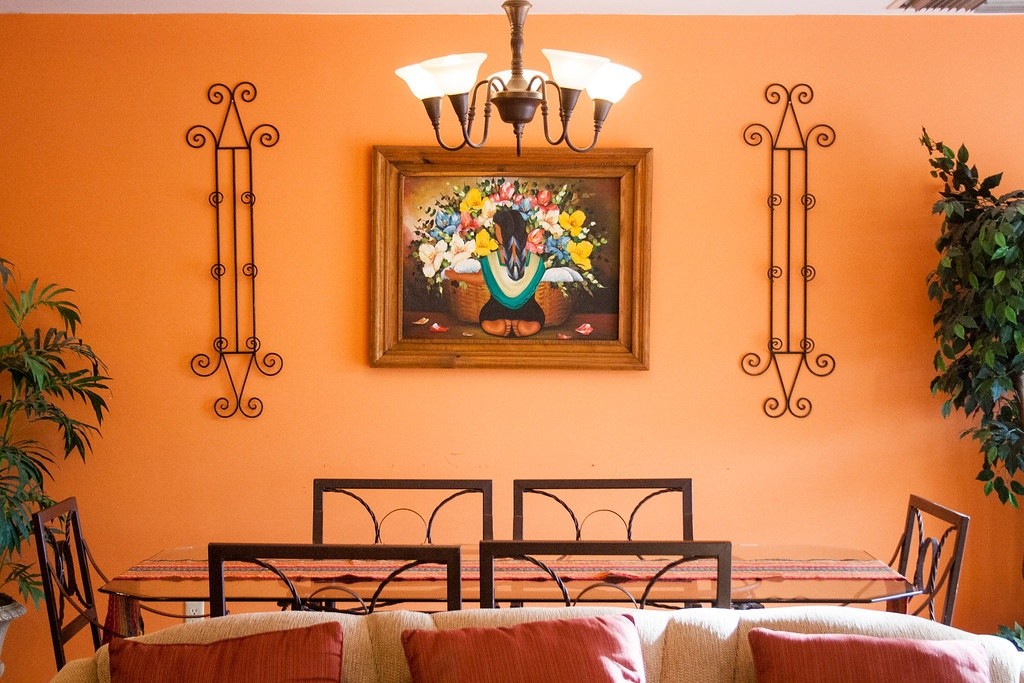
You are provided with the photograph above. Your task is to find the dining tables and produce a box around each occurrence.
[98,546,924,603]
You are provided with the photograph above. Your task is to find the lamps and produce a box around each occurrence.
[394,0,642,158]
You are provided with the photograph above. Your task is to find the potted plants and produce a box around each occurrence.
[0,258,114,678]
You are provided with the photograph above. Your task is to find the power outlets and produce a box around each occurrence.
[185,601,205,623]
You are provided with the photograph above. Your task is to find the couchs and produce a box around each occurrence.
[48,606,1024,682]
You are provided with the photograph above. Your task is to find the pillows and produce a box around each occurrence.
[748,627,989,683]
[401,614,647,683]
[109,621,344,683]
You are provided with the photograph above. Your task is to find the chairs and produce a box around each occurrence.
[32,495,229,673]
[208,478,732,618]
[836,494,970,625]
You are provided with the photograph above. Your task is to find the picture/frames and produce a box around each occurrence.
[368,145,653,371]
